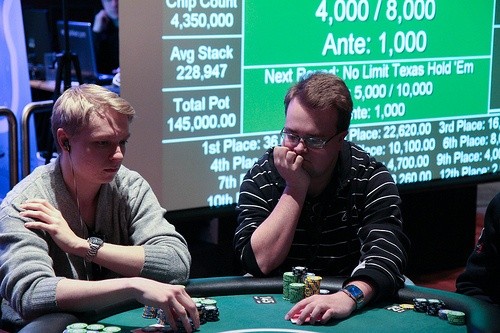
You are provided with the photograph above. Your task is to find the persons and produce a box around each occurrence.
[0,83,202,333]
[233,71,411,325]
[92,0,120,76]
[455,193,500,333]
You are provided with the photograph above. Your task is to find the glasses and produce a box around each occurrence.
[280,127,345,149]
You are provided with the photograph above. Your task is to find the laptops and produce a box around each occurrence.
[54,20,116,82]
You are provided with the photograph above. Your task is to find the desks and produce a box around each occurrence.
[30,80,76,94]
[16,276,500,333]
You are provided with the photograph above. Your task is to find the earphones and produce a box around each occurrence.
[63,138,71,152]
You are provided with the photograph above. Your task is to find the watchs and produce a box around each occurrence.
[340,284,368,311]
[85,237,103,264]
